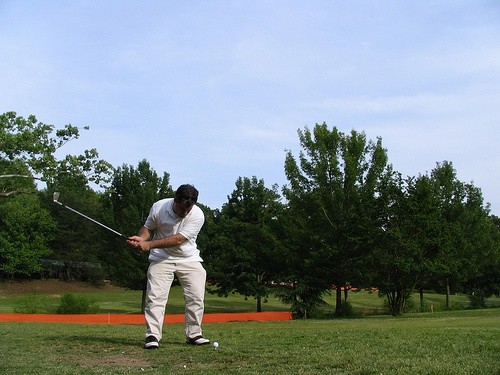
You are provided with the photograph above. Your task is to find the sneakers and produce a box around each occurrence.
[185,335,210,345]
[144,336,159,349]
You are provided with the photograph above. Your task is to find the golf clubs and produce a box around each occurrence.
[52,191,128,242]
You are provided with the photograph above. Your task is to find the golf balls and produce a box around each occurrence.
[212,341,219,348]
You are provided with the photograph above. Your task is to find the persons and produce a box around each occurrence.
[125,184,211,349]
